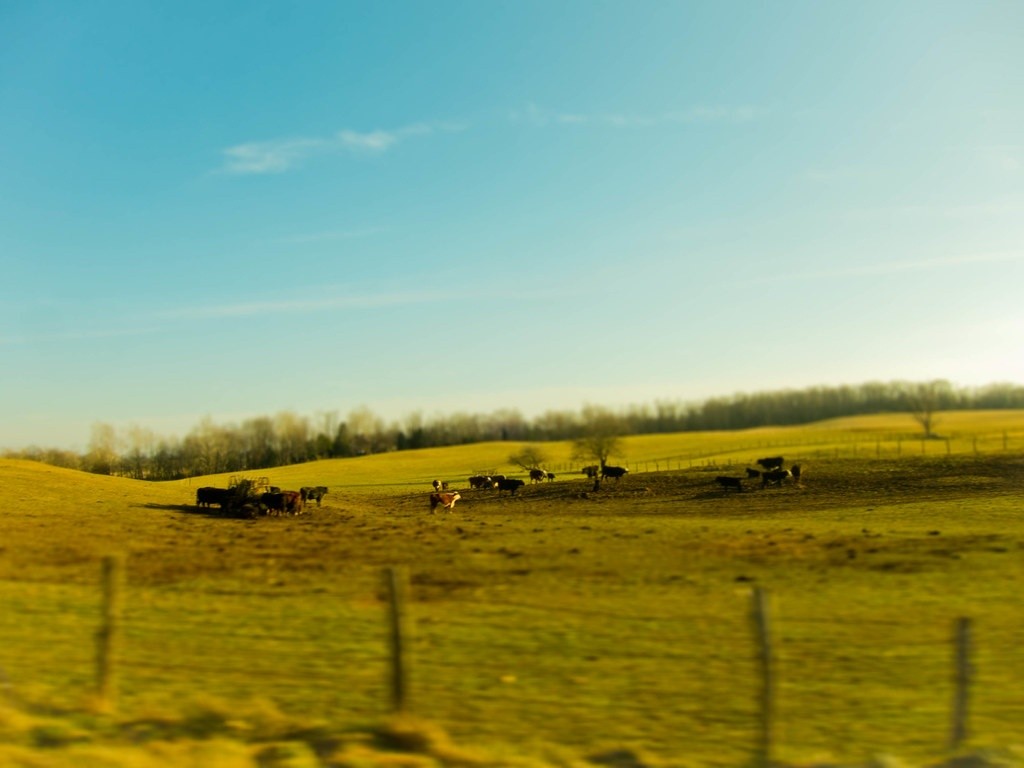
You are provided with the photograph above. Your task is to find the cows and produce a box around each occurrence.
[745,456,808,488]
[196,479,330,520]
[429,469,556,515]
[581,464,630,484]
